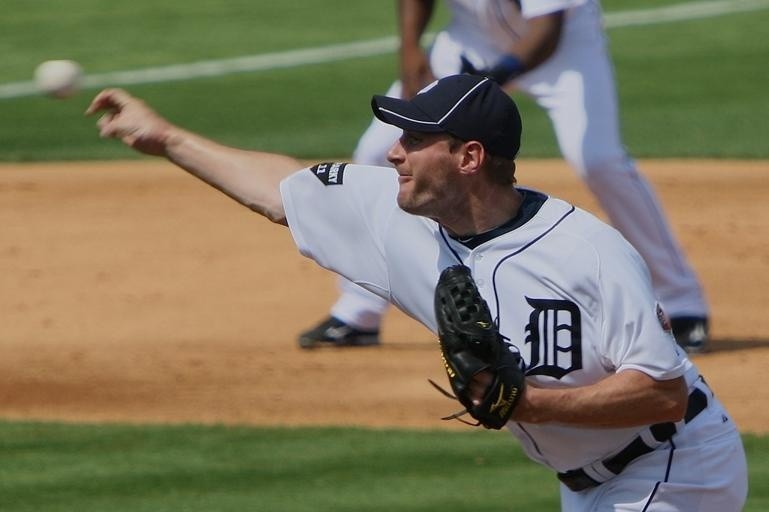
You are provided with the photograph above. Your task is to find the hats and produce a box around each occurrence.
[371,73,522,160]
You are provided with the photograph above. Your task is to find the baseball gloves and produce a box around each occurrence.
[434,264,524,431]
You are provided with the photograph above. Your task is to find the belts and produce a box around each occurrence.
[557,374,713,492]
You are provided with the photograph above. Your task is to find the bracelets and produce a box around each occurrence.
[496,53,524,82]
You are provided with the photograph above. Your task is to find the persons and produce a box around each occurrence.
[299,0,710,353]
[85,73,748,512]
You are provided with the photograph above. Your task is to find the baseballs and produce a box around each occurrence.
[34,59,82,99]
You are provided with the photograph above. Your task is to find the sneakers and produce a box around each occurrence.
[300,315,378,349]
[670,315,708,352]
